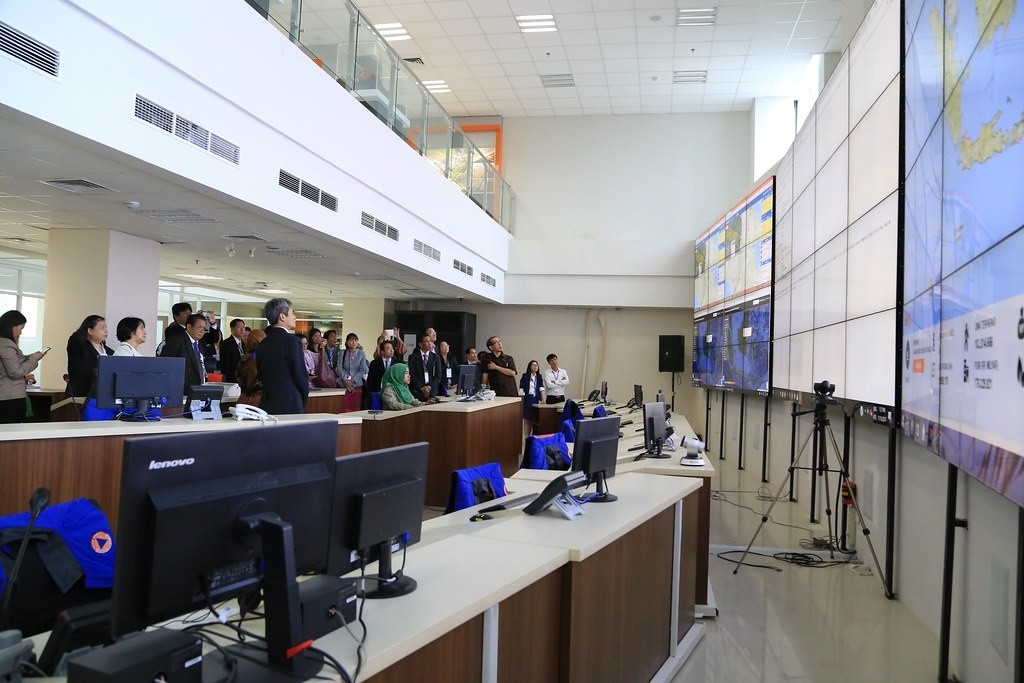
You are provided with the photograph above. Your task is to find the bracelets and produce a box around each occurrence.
[210,322,216,325]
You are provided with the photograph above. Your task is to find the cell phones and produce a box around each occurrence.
[202,311,210,318]
[42,347,51,354]
[385,330,394,336]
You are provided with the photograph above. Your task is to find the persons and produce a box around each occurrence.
[164,302,192,340]
[519,360,545,439]
[0,310,47,423]
[336,332,370,412]
[367,327,408,410]
[323,330,341,370]
[480,336,519,397]
[544,354,569,405]
[160,315,206,386]
[408,327,442,402]
[255,298,309,415]
[463,346,487,365]
[197,310,223,373]
[307,328,321,353]
[437,341,458,390]
[221,319,251,383]
[112,317,147,356]
[295,334,317,389]
[379,363,435,411]
[65,315,115,399]
[235,329,268,409]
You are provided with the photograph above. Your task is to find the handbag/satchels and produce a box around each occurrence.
[25,391,35,418]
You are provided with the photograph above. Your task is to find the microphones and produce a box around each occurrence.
[0,486,52,675]
[63,374,82,422]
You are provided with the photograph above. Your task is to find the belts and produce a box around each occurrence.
[548,395,563,398]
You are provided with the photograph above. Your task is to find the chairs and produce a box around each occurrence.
[561,399,584,427]
[520,432,571,471]
[0,498,117,641]
[592,405,608,418]
[82,397,160,421]
[561,420,576,443]
[442,462,509,515]
[370,391,384,410]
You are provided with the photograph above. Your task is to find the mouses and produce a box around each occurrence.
[470,514,493,522]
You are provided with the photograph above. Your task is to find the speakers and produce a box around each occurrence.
[659,334,685,374]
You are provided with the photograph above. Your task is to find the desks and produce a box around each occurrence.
[0,386,718,683]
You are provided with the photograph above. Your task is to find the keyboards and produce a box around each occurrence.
[479,493,540,513]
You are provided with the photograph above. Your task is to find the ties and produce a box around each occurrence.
[193,343,201,364]
[384,360,388,370]
[423,353,429,369]
[238,342,242,354]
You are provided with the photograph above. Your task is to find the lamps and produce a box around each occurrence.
[248,244,256,257]
[226,242,236,257]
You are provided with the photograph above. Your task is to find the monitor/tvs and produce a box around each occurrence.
[457,365,476,401]
[601,382,608,406]
[98,355,185,422]
[112,418,338,683]
[632,384,671,459]
[571,416,621,503]
[326,440,429,599]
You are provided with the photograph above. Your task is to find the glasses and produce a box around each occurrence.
[491,339,503,345]
[192,325,208,334]
[531,364,540,367]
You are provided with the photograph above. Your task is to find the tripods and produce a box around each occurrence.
[733,396,895,602]
